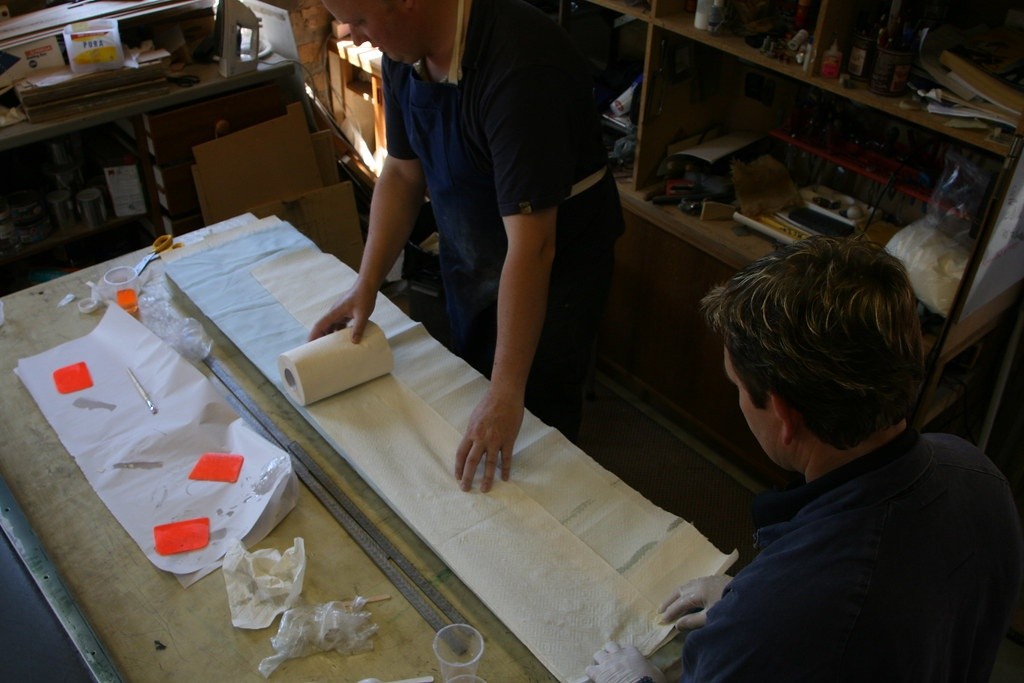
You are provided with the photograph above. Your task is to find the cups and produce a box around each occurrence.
[693,0,712,30]
[433,623,484,683]
[444,674,487,683]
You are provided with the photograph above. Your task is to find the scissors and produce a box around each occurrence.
[135,234,186,276]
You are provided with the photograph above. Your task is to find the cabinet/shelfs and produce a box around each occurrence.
[559,0,1024,493]
[0,52,295,296]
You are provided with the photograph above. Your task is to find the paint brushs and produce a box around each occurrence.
[125,366,158,415]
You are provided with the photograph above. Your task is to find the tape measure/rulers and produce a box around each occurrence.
[201,351,480,655]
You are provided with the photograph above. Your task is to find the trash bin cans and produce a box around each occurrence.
[403,199,453,349]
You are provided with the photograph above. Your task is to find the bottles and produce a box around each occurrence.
[707,0,727,38]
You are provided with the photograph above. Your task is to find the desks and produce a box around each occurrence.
[1,211,735,682]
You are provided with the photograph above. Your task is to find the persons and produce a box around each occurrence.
[585,235,1024,682]
[308,0,627,493]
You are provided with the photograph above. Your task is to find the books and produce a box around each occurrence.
[927,25,1024,128]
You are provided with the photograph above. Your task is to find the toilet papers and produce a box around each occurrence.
[277,319,395,408]
